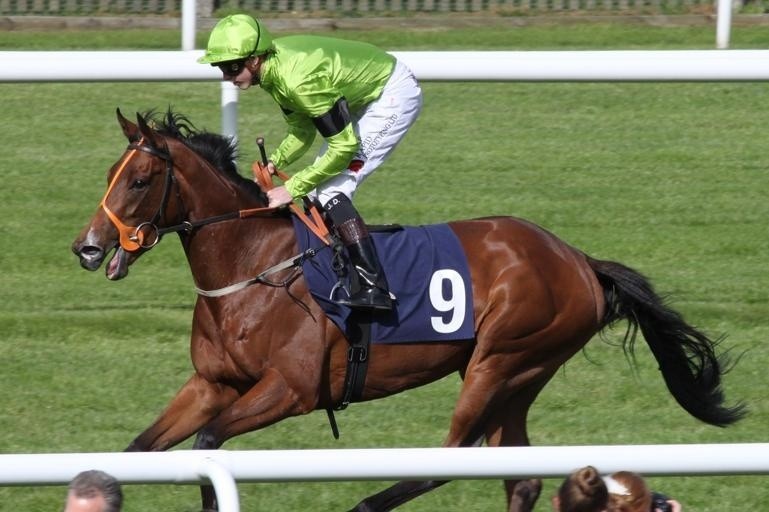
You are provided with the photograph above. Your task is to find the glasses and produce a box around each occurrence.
[219,60,244,76]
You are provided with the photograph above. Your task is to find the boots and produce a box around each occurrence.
[338,219,392,310]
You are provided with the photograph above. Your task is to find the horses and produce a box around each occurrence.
[72,103,762,511]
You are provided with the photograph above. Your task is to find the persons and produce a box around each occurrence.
[602,468,683,512]
[549,463,610,512]
[194,11,426,309]
[60,468,124,511]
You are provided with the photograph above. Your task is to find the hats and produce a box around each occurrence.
[196,13,275,64]
